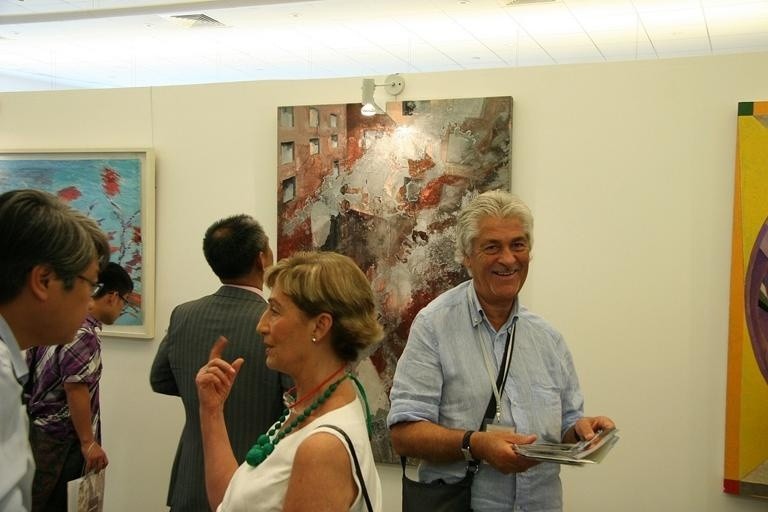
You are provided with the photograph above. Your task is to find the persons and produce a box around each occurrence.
[148,214,296,511]
[195,252,382,511]
[384,190,615,512]
[0,189,110,511]
[20,261,134,511]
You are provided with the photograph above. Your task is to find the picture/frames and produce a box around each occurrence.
[0,147,155,339]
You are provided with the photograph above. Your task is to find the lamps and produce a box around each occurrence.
[360,76,404,117]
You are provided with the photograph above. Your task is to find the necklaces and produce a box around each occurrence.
[244,370,373,469]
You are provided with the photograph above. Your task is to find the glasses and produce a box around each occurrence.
[120,294,131,309]
[77,273,106,298]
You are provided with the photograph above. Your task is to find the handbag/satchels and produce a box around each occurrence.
[401,476,475,510]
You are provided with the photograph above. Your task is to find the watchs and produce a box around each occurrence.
[460,429,481,466]
